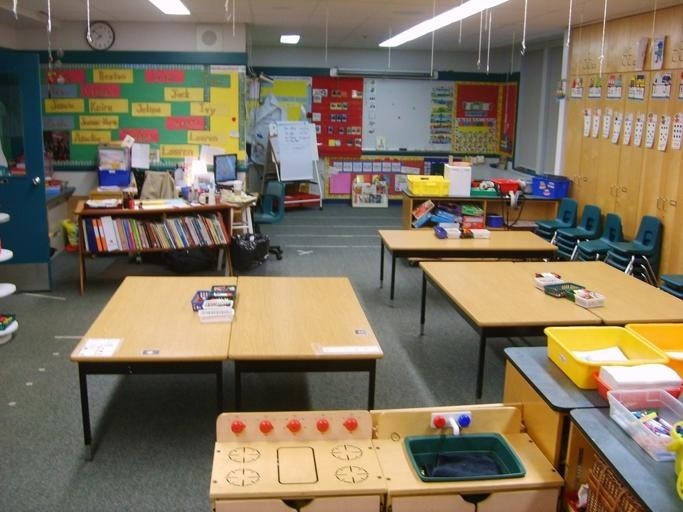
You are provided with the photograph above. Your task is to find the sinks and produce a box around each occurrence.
[403,431,527,481]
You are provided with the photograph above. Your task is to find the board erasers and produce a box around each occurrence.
[399,147,407,151]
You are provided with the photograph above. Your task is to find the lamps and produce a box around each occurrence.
[376,1,515,50]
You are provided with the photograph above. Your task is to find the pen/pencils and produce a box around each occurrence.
[631,407,679,437]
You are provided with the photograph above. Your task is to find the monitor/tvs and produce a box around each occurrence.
[213,154,238,184]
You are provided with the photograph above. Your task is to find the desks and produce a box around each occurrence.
[378,229,560,300]
[69,276,238,461]
[225,276,383,411]
[418,261,683,400]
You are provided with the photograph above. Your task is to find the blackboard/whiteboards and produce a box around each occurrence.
[361,75,455,156]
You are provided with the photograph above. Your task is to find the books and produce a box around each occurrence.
[82,213,229,253]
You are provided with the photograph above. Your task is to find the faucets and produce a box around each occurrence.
[449,417,460,437]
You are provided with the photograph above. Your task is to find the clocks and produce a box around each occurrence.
[82,19,115,53]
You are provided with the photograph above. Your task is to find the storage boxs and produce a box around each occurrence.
[530,176,570,198]
[405,173,450,195]
[98,167,131,187]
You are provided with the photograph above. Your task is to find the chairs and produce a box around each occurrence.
[533,193,662,288]
[250,181,290,261]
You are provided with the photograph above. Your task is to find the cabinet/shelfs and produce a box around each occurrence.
[504,346,681,512]
[208,404,564,512]
[62,188,252,298]
[399,186,561,233]
